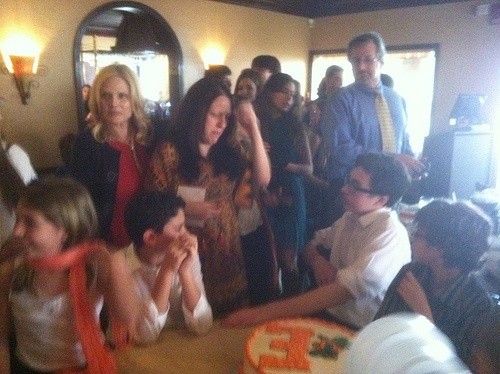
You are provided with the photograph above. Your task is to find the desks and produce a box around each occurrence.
[110,310,254,374]
[420,131,497,200]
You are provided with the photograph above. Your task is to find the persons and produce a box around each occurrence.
[59,33,430,329]
[105,190,213,348]
[0,175,136,374]
[0,140,39,251]
[372,197,494,363]
[470,308,500,374]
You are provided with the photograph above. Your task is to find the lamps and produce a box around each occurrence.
[0,54,49,106]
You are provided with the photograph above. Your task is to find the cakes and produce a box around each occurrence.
[237,316,357,374]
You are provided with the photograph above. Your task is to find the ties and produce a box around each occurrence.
[374,90,397,154]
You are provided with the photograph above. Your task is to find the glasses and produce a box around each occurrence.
[410,229,430,243]
[346,180,377,196]
[351,55,377,64]
